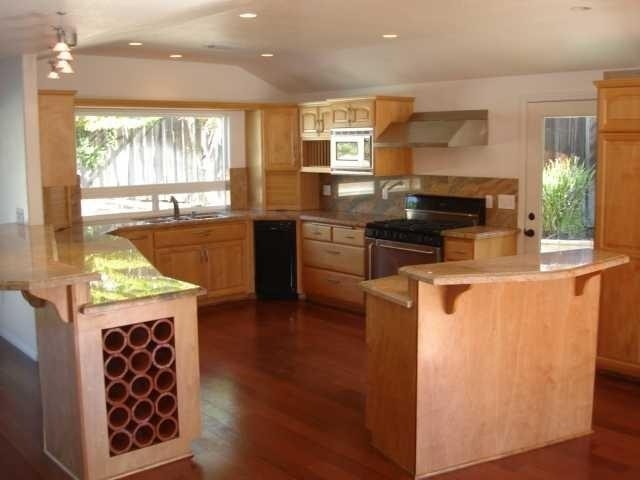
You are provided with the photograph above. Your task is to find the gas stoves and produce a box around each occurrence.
[365,218,473,247]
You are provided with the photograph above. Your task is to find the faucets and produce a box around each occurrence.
[169,196,180,218]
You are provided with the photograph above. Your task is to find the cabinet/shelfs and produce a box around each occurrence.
[299,107,334,138]
[593,79,640,381]
[154,222,248,299]
[302,221,365,313]
[261,108,300,172]
[331,100,375,127]
[443,236,474,262]
[115,230,154,264]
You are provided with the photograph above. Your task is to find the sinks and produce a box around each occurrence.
[134,214,191,229]
[190,212,231,222]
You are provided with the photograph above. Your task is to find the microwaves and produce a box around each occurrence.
[330,134,371,169]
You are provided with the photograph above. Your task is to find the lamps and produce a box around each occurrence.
[47,28,78,79]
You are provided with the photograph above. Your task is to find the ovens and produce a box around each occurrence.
[365,239,442,280]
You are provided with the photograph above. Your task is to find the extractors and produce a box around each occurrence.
[373,110,490,150]
